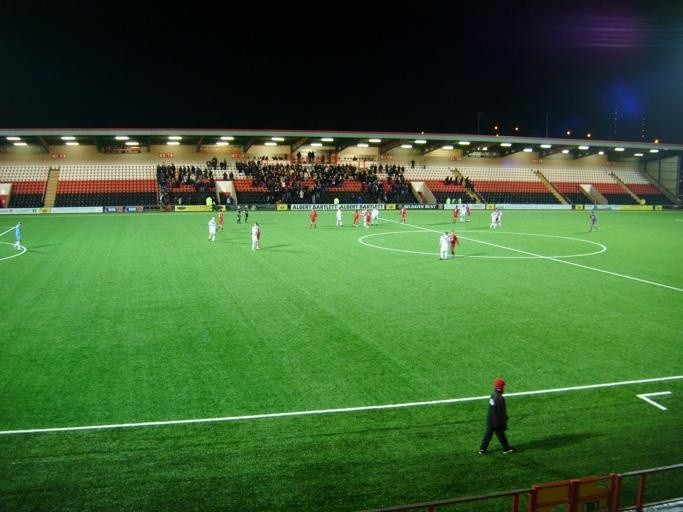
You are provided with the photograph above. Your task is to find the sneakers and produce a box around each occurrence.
[478,448,514,456]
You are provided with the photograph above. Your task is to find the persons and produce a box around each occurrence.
[589,211,600,232]
[155,151,502,258]
[477,378,517,454]
[14,222,22,251]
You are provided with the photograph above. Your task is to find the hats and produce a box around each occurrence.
[495,379,505,389]
